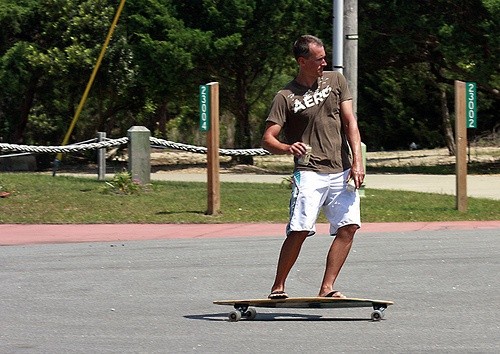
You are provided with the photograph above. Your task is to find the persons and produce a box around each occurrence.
[263,35,366,298]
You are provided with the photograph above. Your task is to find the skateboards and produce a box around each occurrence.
[212,297,394,322]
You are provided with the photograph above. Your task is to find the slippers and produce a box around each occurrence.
[268,290,288,299]
[326,290,346,299]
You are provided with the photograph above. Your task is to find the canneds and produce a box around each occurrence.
[297,144,312,166]
